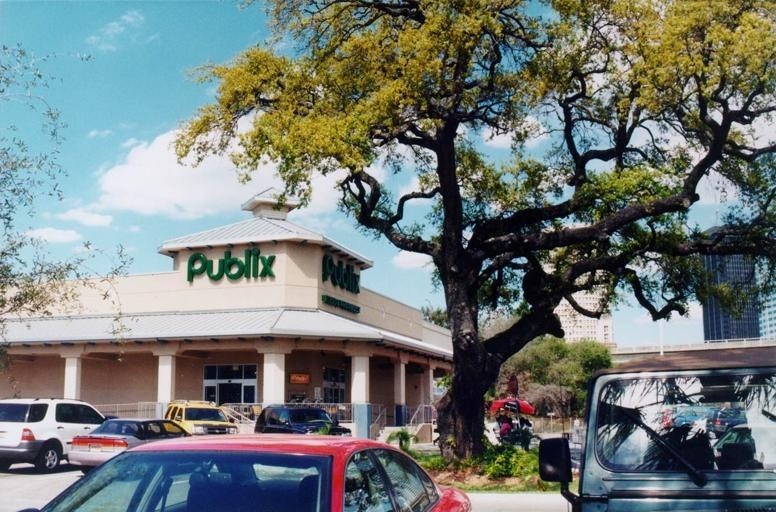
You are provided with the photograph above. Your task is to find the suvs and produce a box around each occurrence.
[254,406,351,437]
[0,396,134,474]
[163,399,240,437]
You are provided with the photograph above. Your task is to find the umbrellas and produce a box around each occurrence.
[487,396,536,416]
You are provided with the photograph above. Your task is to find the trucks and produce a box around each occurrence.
[538,366,776,512]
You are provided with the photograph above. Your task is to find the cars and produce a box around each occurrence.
[68,418,194,474]
[15,434,471,511]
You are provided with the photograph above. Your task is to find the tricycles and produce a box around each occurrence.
[502,430,542,452]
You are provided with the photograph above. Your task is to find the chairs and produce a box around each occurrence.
[493,428,509,445]
[293,475,319,512]
[188,485,233,512]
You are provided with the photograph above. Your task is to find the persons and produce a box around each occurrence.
[433,436,439,445]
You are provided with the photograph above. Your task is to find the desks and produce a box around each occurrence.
[512,431,521,445]
